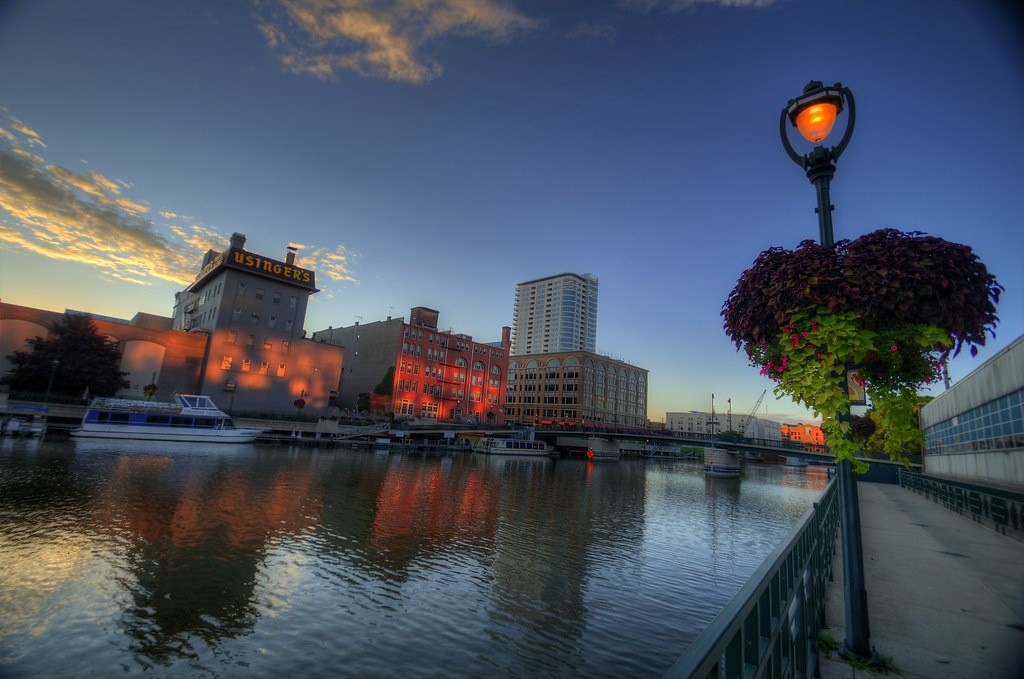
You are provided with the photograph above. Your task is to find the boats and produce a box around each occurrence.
[473,436,555,456]
[5,406,49,435]
[69,391,263,443]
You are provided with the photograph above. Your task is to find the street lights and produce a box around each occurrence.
[777,78,878,665]
[712,393,714,447]
[728,398,731,434]
